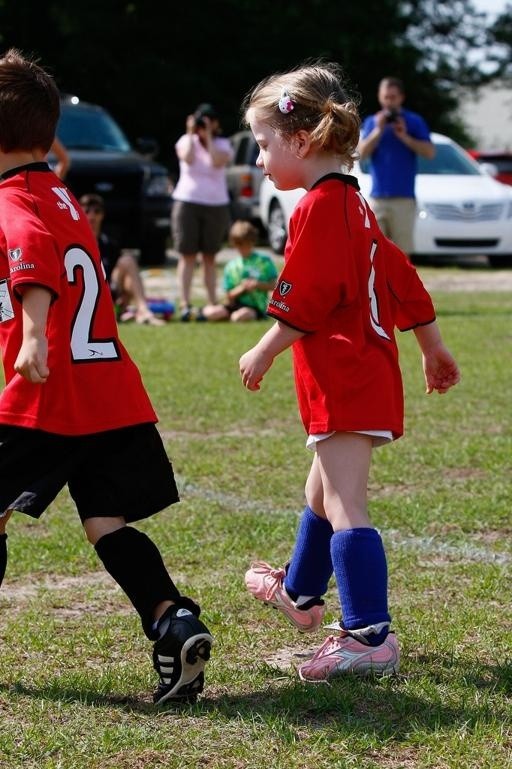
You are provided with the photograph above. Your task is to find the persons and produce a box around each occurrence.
[199,219,276,324]
[168,102,236,323]
[80,192,165,327]
[49,137,73,184]
[238,62,461,683]
[1,48,214,707]
[357,75,434,261]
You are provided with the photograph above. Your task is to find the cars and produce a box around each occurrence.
[48,97,173,264]
[258,130,512,268]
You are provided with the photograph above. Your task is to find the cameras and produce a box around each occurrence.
[194,111,206,132]
[385,105,400,123]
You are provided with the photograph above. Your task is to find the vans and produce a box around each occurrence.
[223,130,265,224]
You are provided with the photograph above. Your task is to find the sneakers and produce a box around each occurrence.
[150,601,214,705]
[298,618,400,685]
[244,559,326,633]
[118,299,207,328]
